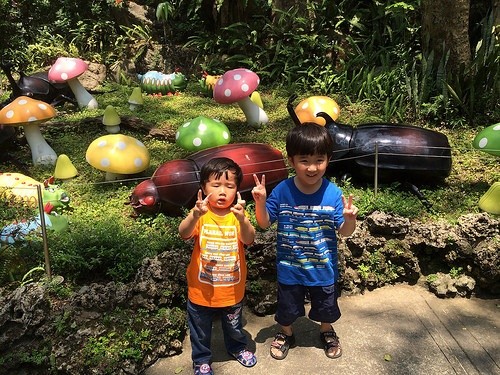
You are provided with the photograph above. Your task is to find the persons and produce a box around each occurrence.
[178,157,257,375]
[252,121,359,360]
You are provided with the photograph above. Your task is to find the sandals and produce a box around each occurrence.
[269,331,296,359]
[320,325,342,358]
[229,347,257,366]
[193,363,213,375]
